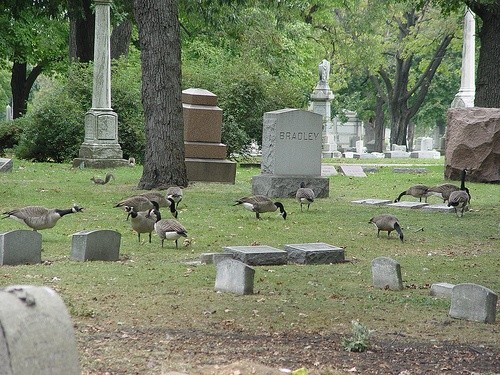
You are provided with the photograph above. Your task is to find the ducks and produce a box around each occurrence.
[393,184,433,204]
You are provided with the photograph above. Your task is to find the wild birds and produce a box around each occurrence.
[125,206,157,244]
[428,183,459,203]
[446,167,472,219]
[112,197,159,212]
[367,214,405,243]
[232,194,288,221]
[1,203,84,231]
[89,172,114,185]
[166,186,183,207]
[145,208,188,249]
[295,181,315,212]
[131,192,178,220]
